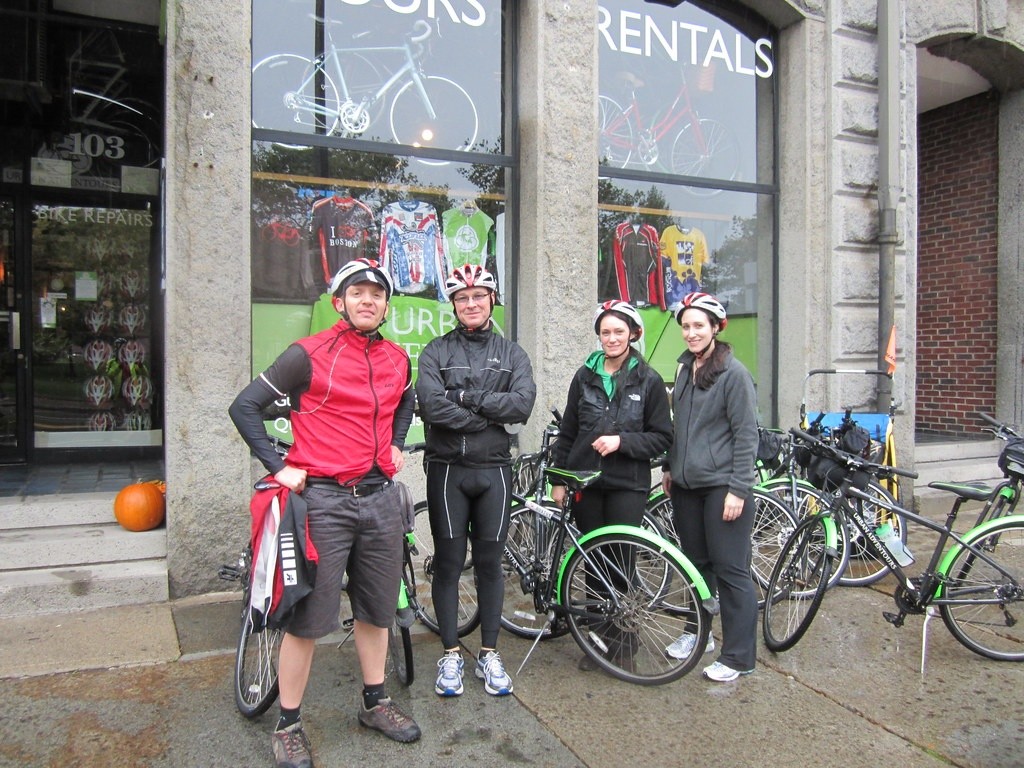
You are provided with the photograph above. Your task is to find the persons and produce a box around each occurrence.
[662,291,759,682]
[414,263,537,696]
[229,259,422,768]
[549,299,674,670]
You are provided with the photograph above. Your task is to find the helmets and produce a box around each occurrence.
[593,299,643,343]
[674,292,727,332]
[85,231,154,432]
[330,256,393,303]
[444,264,498,299]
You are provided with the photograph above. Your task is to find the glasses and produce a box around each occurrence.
[454,290,491,303]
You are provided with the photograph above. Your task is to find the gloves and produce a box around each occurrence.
[444,389,463,407]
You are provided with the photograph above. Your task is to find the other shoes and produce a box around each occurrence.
[579,650,611,670]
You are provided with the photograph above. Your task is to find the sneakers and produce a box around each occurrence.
[703,660,755,681]
[358,689,421,742]
[271,717,311,768]
[664,632,715,659]
[434,651,465,696]
[475,650,513,695]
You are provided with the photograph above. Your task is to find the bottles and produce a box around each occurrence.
[876,523,914,567]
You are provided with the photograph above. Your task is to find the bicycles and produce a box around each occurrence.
[252,13,480,167]
[762,427,1024,676]
[949,411,1024,596]
[501,408,908,636]
[337,528,419,688]
[219,438,281,717]
[599,61,741,198]
[397,441,716,683]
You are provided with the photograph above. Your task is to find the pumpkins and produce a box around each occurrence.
[114,478,165,532]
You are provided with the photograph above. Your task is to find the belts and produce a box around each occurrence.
[304,480,392,498]
